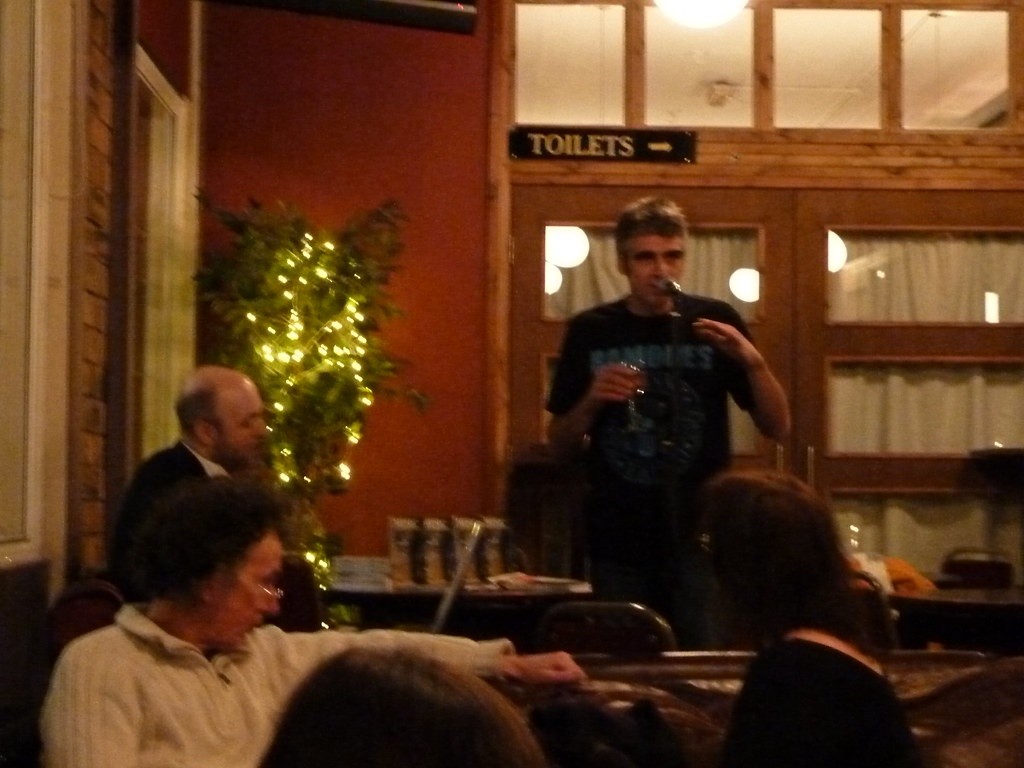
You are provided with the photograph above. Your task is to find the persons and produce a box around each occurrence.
[107,365,268,596]
[699,470,926,768]
[39,474,588,768]
[253,647,552,768]
[545,195,792,646]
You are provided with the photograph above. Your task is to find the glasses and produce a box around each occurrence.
[256,582,284,601]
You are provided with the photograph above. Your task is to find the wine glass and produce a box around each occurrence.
[613,361,645,433]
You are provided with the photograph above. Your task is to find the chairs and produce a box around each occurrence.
[844,568,900,650]
[558,679,729,748]
[263,555,320,632]
[44,576,124,646]
[536,600,678,653]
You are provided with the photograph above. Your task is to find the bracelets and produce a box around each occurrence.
[742,358,765,371]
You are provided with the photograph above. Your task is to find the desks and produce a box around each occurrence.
[321,576,594,639]
[889,588,1024,621]
